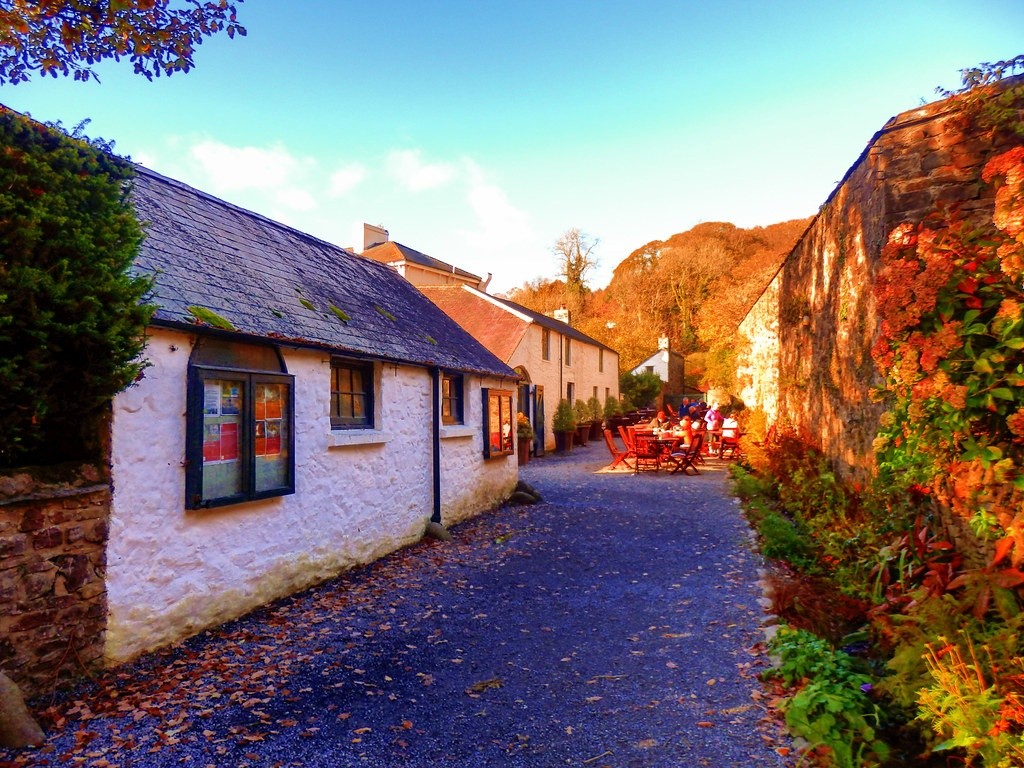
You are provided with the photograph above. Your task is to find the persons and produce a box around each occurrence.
[679,396,706,419]
[722,412,743,458]
[647,409,673,430]
[673,416,694,448]
[703,402,727,454]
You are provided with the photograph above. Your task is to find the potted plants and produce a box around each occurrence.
[587,397,604,441]
[517,412,535,466]
[573,399,593,447]
[551,399,578,451]
[603,396,638,438]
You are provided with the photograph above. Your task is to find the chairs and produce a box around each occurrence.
[604,403,748,476]
